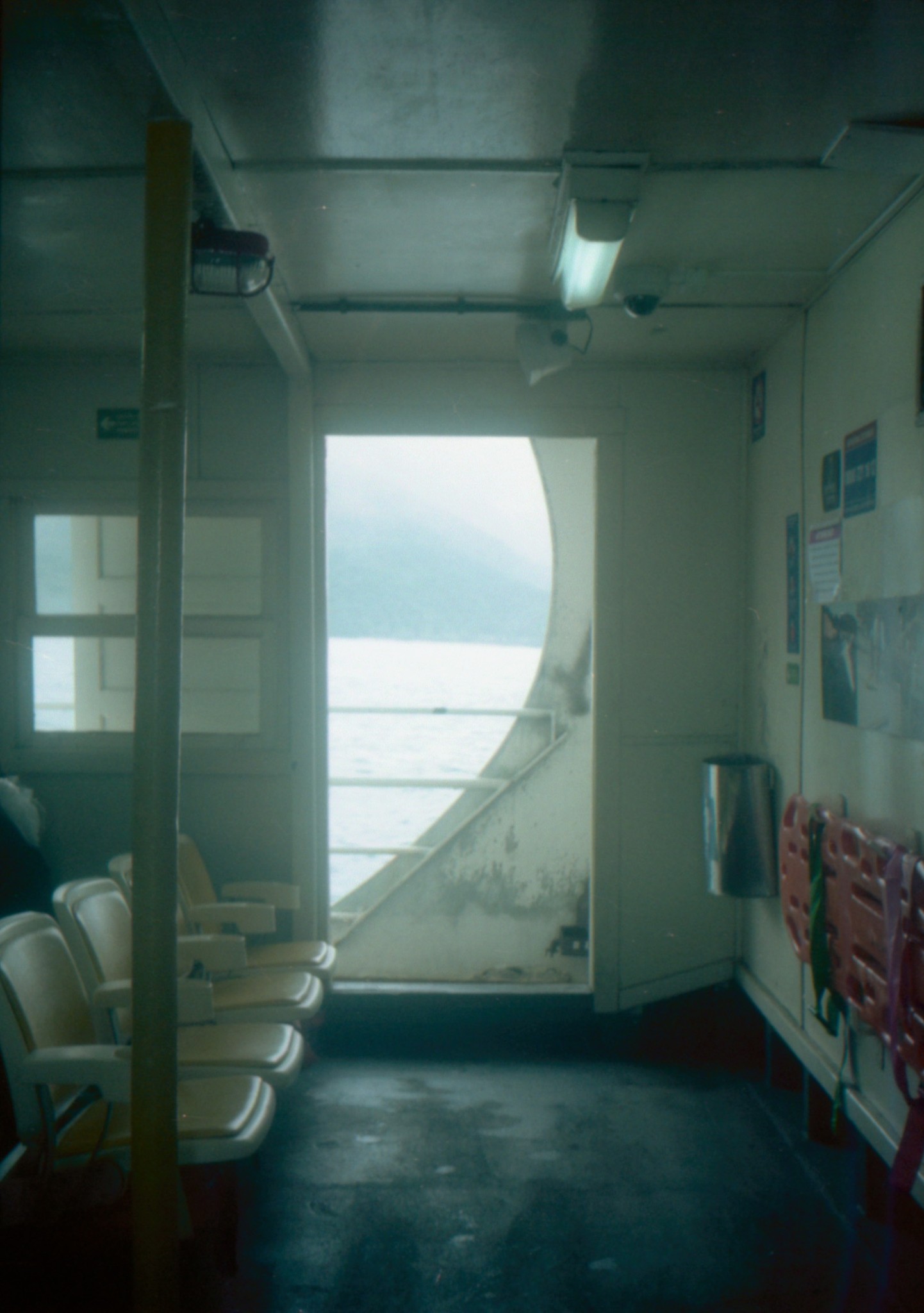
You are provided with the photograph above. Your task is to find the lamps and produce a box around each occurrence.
[516,153,650,383]
[192,215,275,298]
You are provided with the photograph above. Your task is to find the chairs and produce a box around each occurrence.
[0,831,337,1281]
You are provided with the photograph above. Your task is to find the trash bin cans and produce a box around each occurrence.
[702,750,776,898]
